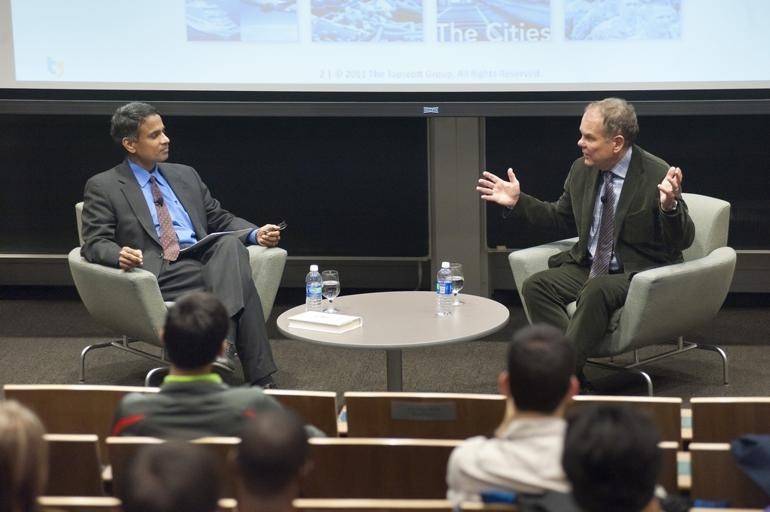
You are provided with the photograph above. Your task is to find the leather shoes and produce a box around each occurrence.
[211,338,237,377]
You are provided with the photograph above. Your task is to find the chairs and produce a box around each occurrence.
[68,201,290,387]
[509,192,737,397]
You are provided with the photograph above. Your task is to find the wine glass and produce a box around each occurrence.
[451,264,465,307]
[322,271,340,314]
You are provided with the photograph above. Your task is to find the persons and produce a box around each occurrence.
[226,408,315,512]
[112,290,325,437]
[562,406,660,511]
[80,102,282,388]
[445,323,670,503]
[475,97,695,376]
[1,393,48,511]
[116,440,219,511]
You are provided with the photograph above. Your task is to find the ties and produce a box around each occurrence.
[150,176,180,262]
[589,172,614,279]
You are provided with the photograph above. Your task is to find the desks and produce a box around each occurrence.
[274,288,511,392]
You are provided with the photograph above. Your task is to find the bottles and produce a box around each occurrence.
[305,264,322,312]
[435,261,453,314]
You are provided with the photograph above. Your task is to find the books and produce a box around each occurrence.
[288,310,363,334]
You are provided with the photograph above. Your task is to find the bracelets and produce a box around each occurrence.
[660,199,678,213]
